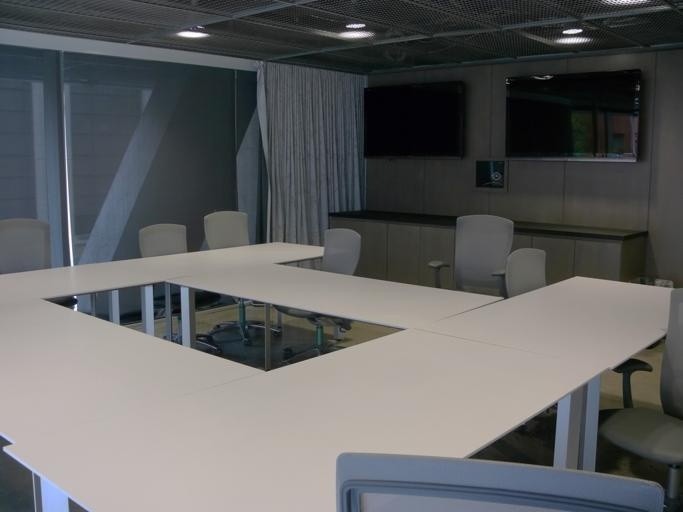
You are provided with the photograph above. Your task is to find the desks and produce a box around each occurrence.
[0,242,682,512]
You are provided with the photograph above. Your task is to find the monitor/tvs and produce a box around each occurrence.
[504,67,644,166]
[363,79,469,163]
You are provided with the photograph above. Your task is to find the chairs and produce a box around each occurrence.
[137,221,221,354]
[202,210,282,347]
[427,215,514,302]
[2,218,81,311]
[273,225,363,362]
